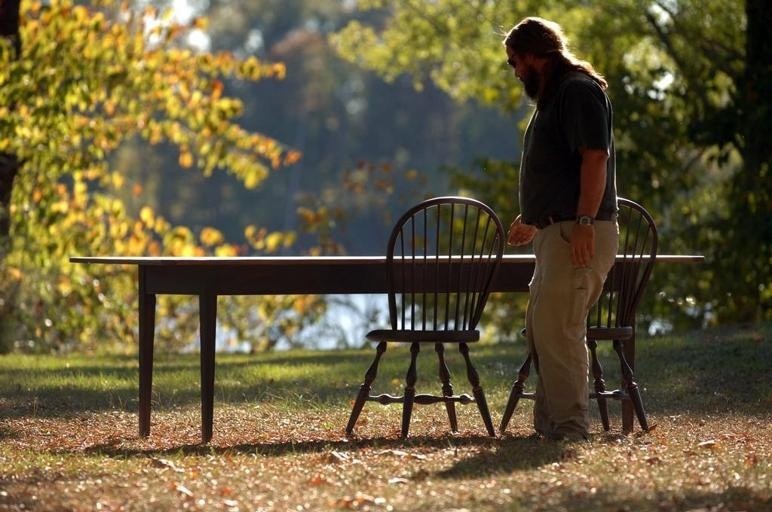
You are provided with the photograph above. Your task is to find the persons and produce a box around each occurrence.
[494,17,620,446]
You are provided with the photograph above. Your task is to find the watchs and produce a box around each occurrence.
[577,215,594,226]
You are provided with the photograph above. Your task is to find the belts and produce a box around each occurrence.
[536,210,612,229]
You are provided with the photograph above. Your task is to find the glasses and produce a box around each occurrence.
[508,53,517,68]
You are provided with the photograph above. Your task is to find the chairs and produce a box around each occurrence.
[498,196,660,433]
[345,197,506,441]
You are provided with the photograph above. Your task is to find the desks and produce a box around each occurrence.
[69,251,706,447]
